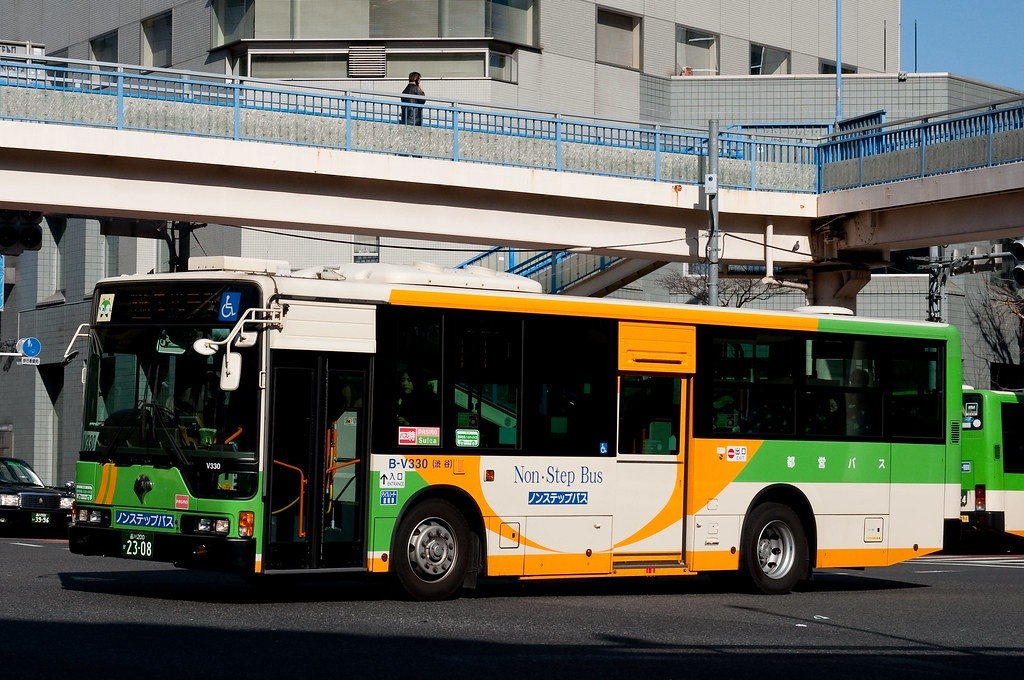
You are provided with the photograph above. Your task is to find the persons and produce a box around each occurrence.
[398,72,426,158]
[828,369,881,436]
[148,372,246,450]
[389,367,437,454]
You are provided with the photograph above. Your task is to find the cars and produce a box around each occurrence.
[0,456,74,537]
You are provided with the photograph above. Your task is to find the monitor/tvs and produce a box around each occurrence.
[180,416,205,433]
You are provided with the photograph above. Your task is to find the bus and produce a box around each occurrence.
[57,256,963,604]
[945,388,1024,557]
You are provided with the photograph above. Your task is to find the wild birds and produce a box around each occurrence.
[791,240,800,252]
